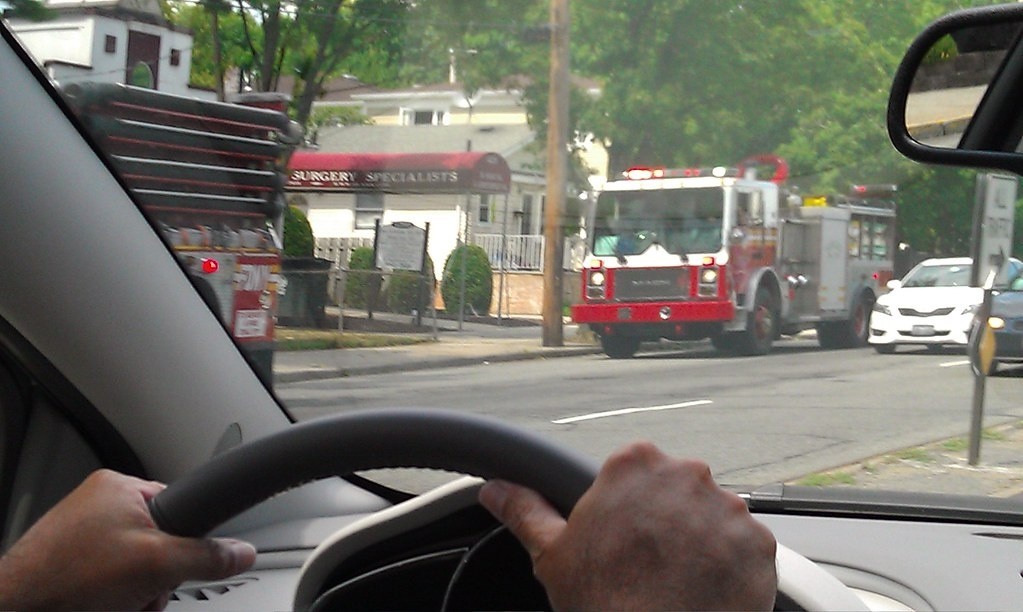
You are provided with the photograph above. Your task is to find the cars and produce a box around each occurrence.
[964,256,1023,378]
[867,257,1023,355]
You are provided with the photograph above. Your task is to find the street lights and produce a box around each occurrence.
[448,44,479,89]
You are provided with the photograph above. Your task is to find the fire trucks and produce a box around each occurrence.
[46,77,320,411]
[567,156,903,362]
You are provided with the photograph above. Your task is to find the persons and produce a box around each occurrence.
[0,440,779,612]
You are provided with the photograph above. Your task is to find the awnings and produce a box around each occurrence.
[285,143,512,329]
